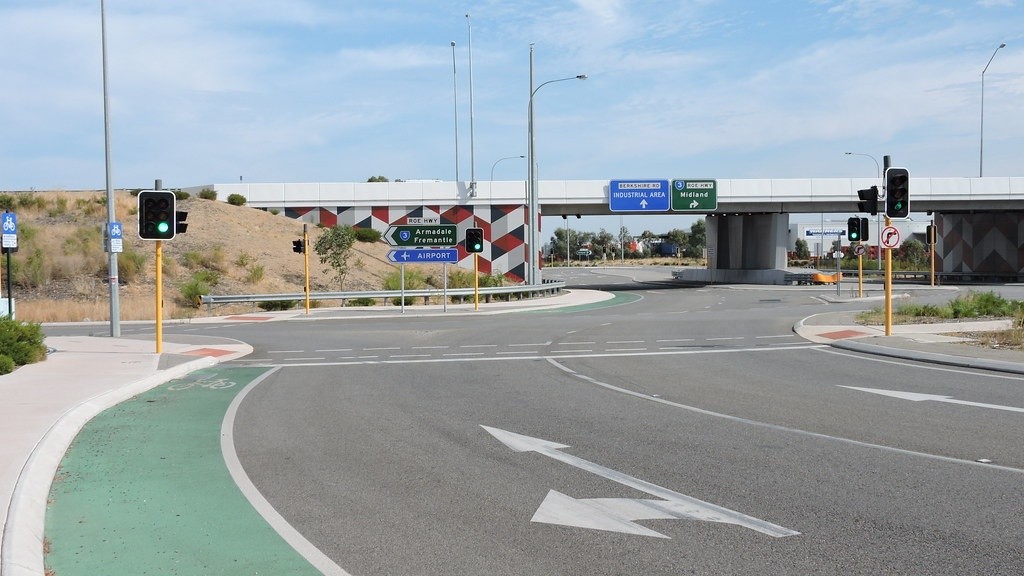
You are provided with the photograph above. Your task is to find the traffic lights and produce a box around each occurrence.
[138,189,176,240]
[465,227,483,253]
[603,247,606,253]
[612,247,615,252]
[176,211,189,234]
[886,167,911,219]
[293,239,305,253]
[848,217,861,241]
[857,185,879,215]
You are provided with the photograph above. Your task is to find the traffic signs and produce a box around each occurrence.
[384,224,458,264]
[671,178,718,211]
[608,179,671,212]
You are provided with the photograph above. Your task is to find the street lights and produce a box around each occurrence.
[450,13,476,182]
[490,155,526,182]
[525,72,590,286]
[978,42,1007,177]
[844,152,880,178]
